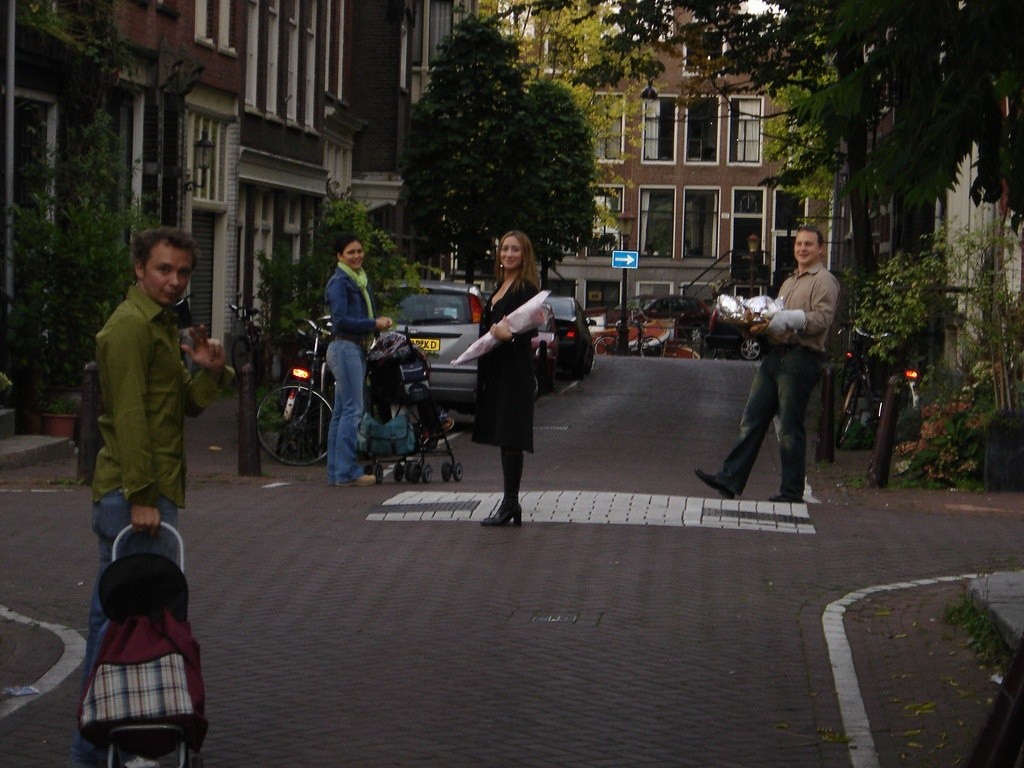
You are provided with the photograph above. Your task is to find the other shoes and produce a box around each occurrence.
[334,474,377,486]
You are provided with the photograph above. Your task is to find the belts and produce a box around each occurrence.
[330,335,362,345]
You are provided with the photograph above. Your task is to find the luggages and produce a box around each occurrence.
[76,521,210,768]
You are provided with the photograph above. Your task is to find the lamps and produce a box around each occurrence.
[640,79,659,104]
[182,126,216,195]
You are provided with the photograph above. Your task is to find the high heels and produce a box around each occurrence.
[481,496,523,526]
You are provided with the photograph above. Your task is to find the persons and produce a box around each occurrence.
[69,227,236,768]
[471,230,539,527]
[694,227,841,503]
[326,235,454,487]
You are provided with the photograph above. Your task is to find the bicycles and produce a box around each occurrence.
[594,320,663,358]
[255,314,334,466]
[228,303,267,390]
[835,323,894,451]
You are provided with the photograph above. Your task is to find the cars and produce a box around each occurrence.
[388,280,486,415]
[606,293,710,332]
[546,297,596,380]
[705,304,763,360]
[482,291,560,395]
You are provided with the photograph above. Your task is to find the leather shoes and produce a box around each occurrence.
[768,491,802,502]
[693,466,736,499]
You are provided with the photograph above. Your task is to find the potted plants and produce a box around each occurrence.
[256,379,285,460]
[39,397,79,438]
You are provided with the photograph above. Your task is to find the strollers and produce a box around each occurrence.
[364,325,464,483]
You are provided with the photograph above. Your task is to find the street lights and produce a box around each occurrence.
[746,233,758,297]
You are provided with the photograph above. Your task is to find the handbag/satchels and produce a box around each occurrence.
[353,410,417,458]
